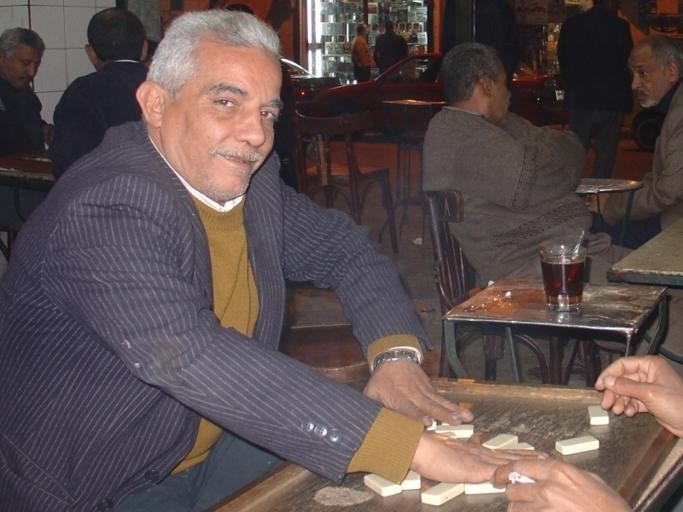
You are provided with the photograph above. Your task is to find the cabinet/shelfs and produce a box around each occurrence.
[320,1,428,86]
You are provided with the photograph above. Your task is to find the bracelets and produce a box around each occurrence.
[372,348,418,369]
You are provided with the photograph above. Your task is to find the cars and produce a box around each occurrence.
[308,46,570,139]
[627,103,668,150]
[143,37,161,65]
[279,56,342,114]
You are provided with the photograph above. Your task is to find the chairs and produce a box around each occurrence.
[420,189,503,382]
[0,98,683,363]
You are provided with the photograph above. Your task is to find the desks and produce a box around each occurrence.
[204,376,681,510]
[443,275,667,385]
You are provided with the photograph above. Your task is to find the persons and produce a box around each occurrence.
[422,41,683,377]
[557,0,634,176]
[373,21,408,75]
[0,8,549,510]
[50,6,149,179]
[0,26,53,233]
[488,353,683,512]
[144,40,157,69]
[351,24,376,83]
[584,33,683,249]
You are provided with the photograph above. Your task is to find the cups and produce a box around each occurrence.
[538,244,586,310]
